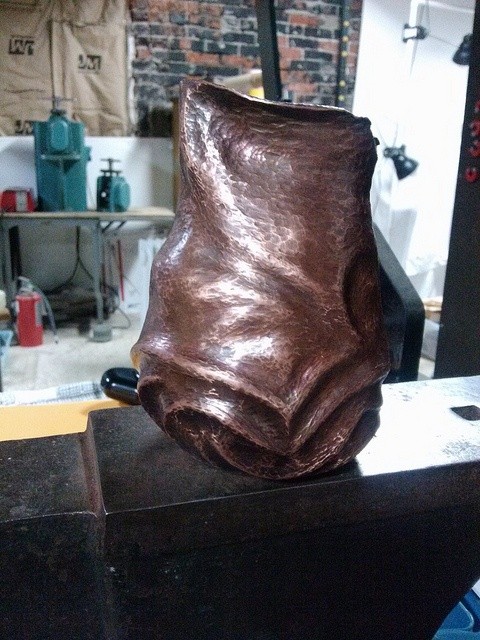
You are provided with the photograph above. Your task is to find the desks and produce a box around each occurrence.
[0,207,176,322]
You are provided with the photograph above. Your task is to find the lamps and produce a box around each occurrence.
[382,146,419,184]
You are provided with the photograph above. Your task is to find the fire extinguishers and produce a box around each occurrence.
[15,276,59,347]
